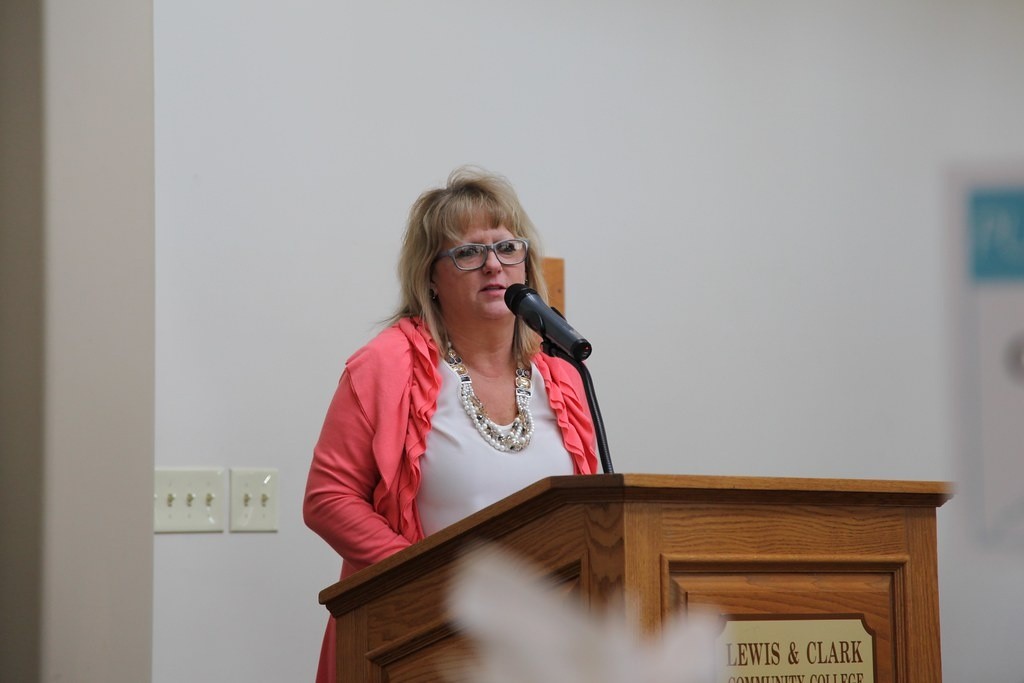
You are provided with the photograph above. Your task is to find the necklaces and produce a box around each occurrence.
[440,339,534,453]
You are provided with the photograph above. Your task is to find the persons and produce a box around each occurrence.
[303,172,599,683]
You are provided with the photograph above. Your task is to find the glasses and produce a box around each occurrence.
[438,238,530,271]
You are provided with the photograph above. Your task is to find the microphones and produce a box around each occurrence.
[504,283,592,360]
